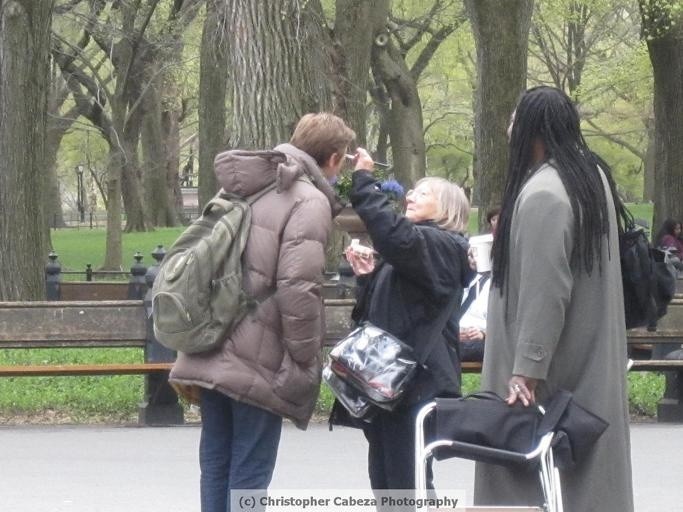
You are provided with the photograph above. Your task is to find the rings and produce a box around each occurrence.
[512,384,520,393]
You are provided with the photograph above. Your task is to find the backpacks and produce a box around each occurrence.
[151,174,317,352]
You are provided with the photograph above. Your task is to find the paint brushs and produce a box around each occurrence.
[345,153,392,167]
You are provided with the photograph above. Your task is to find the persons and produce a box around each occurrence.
[472,87,635,511]
[458,270,490,360]
[485,206,502,237]
[145,110,355,509]
[323,147,474,511]
[655,215,682,262]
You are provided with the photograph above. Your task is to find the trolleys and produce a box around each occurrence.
[405,392,567,512]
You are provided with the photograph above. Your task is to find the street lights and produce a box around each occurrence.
[80,163,84,223]
[73,165,81,214]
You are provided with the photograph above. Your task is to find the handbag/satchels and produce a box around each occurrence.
[620,229,674,332]
[321,321,421,425]
